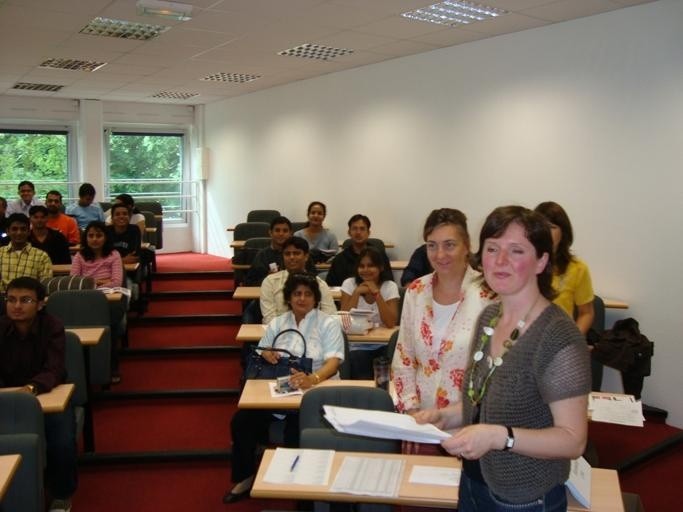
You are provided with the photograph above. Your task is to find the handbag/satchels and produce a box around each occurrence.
[243,330,314,379]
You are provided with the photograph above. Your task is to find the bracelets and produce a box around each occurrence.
[350,293,359,300]
[314,373,321,384]
[372,289,381,300]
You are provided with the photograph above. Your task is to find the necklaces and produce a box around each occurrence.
[466,292,541,405]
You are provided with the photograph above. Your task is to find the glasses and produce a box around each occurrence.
[4,296,39,304]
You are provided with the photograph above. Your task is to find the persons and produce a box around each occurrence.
[294,201,339,262]
[326,216,395,287]
[222,273,345,504]
[406,205,588,510]
[534,201,595,340]
[387,208,500,455]
[260,237,337,324]
[339,248,400,376]
[1,180,144,511]
[397,244,478,322]
[241,215,294,322]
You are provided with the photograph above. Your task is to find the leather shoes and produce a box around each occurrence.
[222,478,252,503]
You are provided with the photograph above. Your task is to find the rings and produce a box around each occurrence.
[297,378,301,382]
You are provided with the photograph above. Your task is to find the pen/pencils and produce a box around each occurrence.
[290,456,300,472]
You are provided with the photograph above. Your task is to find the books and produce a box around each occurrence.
[566,455,592,509]
[317,244,334,263]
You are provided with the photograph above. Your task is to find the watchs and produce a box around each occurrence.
[502,422,514,452]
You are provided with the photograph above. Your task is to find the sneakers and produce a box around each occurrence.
[110,370,122,386]
